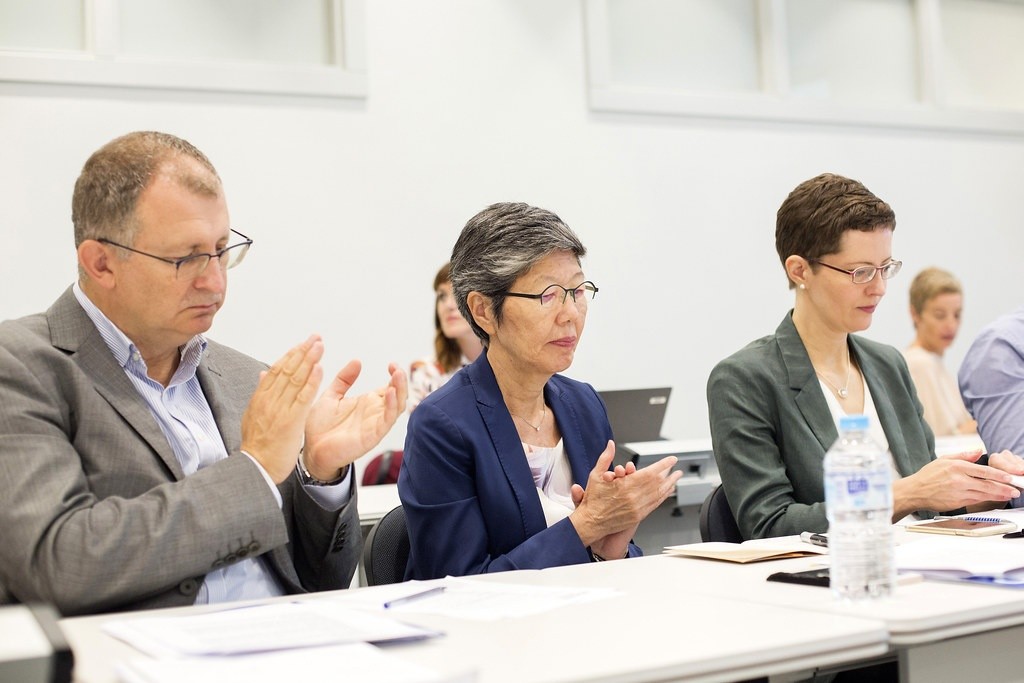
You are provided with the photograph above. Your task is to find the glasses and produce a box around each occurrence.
[799,253,904,285]
[487,282,599,311]
[97,228,254,282]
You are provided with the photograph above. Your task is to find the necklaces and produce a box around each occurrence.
[816,345,850,398]
[509,399,545,431]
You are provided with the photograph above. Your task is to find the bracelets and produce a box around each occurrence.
[298,450,347,486]
[592,545,628,561]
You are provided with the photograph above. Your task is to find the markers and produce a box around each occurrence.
[800,531,828,547]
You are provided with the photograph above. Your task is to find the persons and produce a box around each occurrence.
[407,266,484,410]
[1,128,408,617]
[899,268,982,439]
[397,202,683,584]
[706,172,1024,540]
[958,310,1024,507]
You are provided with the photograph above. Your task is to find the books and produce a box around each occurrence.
[907,517,1018,537]
[662,537,828,563]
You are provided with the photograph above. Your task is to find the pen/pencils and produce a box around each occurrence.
[934,516,1012,524]
[1002,528,1024,539]
[383,587,448,608]
[897,567,973,576]
[1003,567,1024,575]
[958,576,1023,583]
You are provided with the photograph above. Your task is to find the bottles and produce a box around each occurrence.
[823,415,895,615]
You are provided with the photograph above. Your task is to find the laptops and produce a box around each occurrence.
[596,388,671,447]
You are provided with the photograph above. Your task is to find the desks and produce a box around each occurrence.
[61,570,892,683]
[549,507,1024,683]
